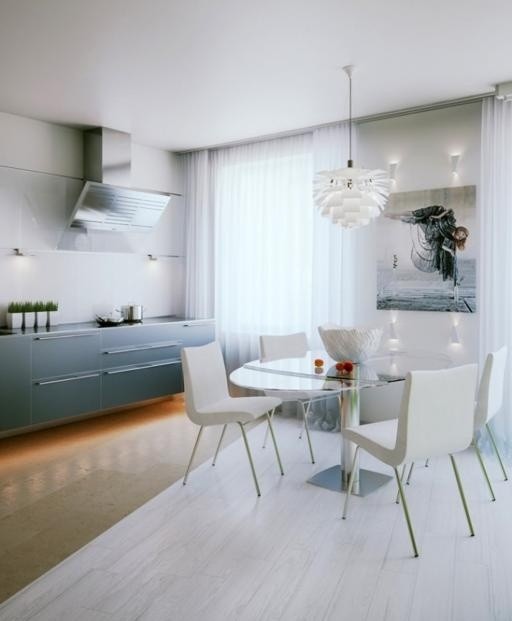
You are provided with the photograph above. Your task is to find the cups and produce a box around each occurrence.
[120,303,143,319]
[99,309,122,322]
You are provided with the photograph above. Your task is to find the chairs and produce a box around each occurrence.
[341,362,475,558]
[180,340,284,496]
[259,332,341,464]
[406,345,511,503]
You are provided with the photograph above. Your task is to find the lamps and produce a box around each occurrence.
[312,64,395,229]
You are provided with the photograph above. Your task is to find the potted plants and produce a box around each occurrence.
[6,301,59,329]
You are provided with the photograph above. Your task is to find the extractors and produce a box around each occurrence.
[65,127,172,234]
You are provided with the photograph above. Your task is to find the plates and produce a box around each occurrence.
[97,320,123,327]
[122,319,142,323]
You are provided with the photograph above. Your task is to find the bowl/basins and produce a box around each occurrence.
[318,326,385,364]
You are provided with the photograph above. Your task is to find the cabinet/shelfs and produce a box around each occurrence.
[0,319,216,439]
